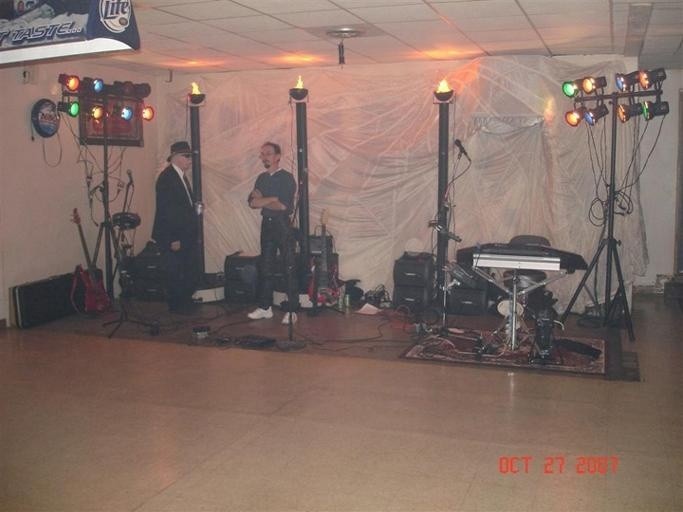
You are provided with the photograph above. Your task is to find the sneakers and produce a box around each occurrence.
[281,312,298,324]
[247,305,274,320]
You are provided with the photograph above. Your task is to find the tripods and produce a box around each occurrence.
[102,185,158,339]
[62,92,144,309]
[557,90,664,342]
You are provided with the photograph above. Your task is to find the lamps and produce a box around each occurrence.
[561,67,670,128]
[58,73,156,122]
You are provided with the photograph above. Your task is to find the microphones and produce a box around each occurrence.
[454,139,471,163]
[125,169,133,186]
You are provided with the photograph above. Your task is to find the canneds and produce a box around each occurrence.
[337,295,343,309]
[344,294,350,307]
[196,202,203,216]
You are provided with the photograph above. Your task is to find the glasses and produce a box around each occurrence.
[181,154,192,158]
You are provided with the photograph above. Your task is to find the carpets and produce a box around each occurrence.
[399,324,607,375]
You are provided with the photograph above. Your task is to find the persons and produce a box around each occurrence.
[243,141,302,326]
[149,140,207,315]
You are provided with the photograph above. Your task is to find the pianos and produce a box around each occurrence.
[455,243,586,273]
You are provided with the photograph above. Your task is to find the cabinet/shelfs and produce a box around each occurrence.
[457,266,568,360]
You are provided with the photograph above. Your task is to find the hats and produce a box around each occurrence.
[167,142,198,161]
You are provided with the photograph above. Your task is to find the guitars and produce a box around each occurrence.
[308,209,340,308]
[71,208,111,312]
